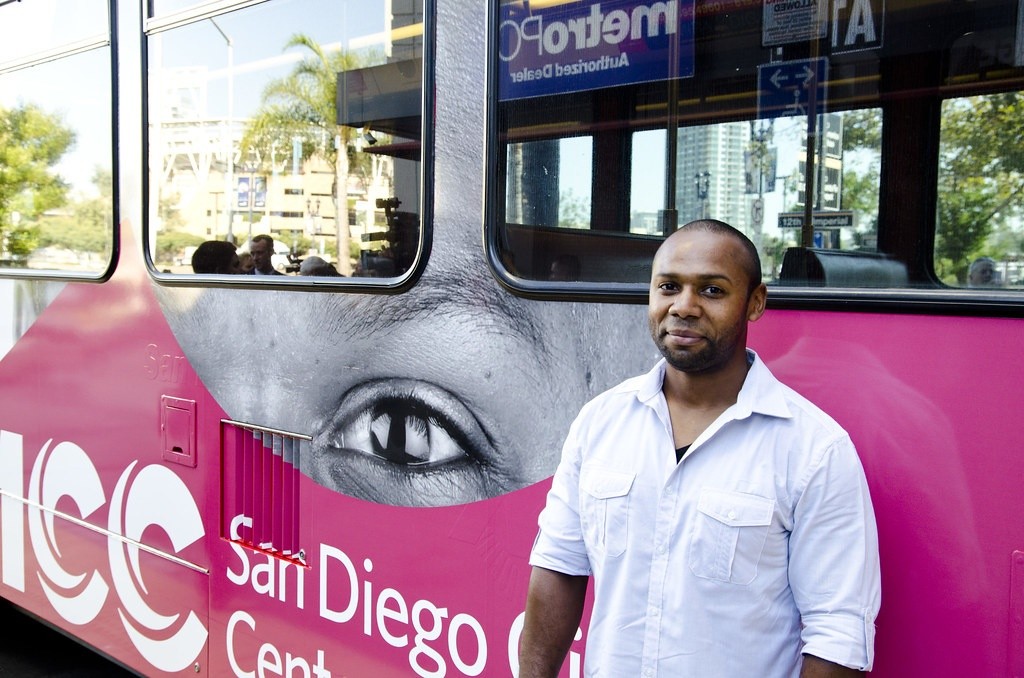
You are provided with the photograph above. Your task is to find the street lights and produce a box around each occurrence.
[306,196,321,257]
[696,171,710,220]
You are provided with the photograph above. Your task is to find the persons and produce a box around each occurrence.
[519,217,881,678]
[191,234,341,276]
[26,0,668,511]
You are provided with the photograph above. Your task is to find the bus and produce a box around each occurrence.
[0,1,1024,678]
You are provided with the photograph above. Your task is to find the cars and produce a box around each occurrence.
[173,244,198,266]
[235,239,291,270]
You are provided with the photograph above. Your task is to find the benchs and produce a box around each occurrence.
[499,223,666,290]
[781,247,909,290]
